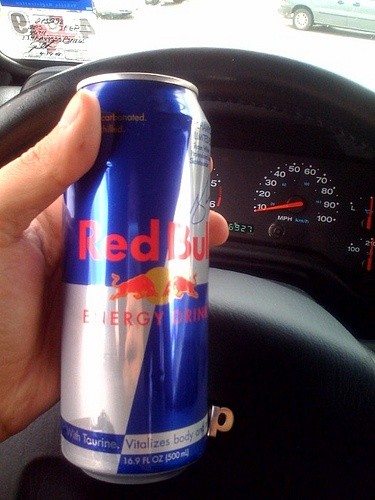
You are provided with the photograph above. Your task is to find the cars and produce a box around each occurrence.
[92,0,133,17]
[278,1,375,33]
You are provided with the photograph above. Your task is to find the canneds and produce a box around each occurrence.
[60,71,211,484]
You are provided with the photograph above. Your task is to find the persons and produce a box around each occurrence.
[1,91,230,450]
